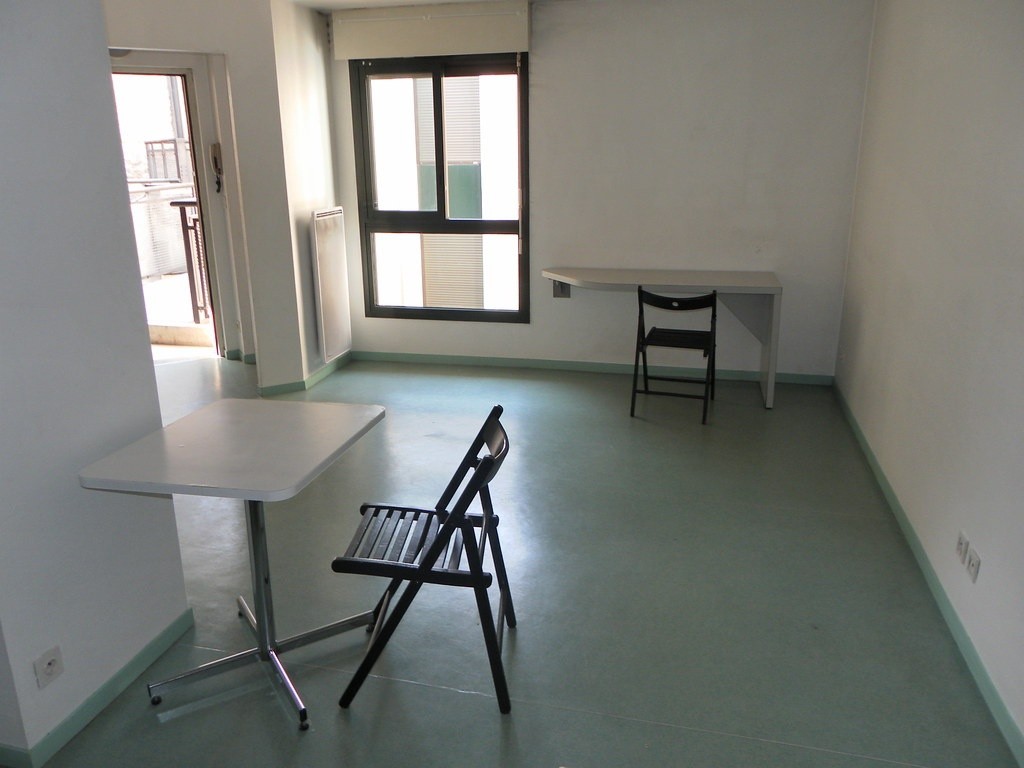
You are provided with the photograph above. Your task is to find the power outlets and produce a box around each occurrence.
[32,644,67,690]
[966,548,980,584]
[954,528,970,564]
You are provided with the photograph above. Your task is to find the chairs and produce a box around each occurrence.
[331,405,518,715]
[630,284,717,425]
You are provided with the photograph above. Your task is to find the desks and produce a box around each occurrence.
[540,267,784,410]
[78,398,387,730]
[169,197,210,324]
